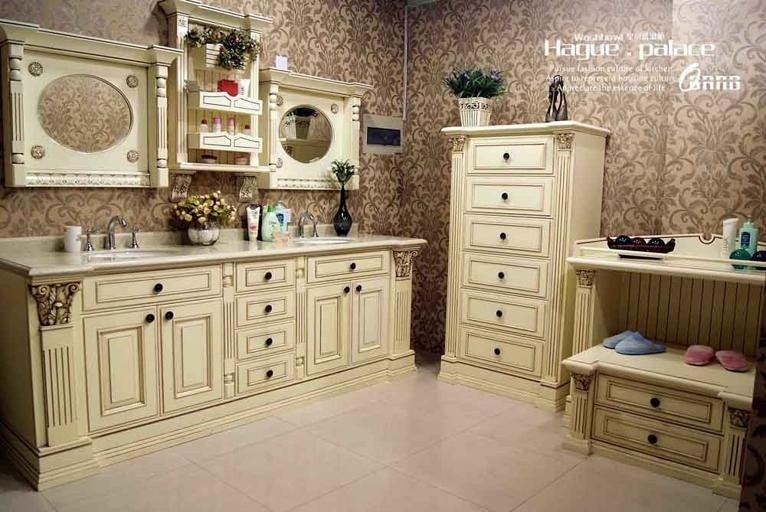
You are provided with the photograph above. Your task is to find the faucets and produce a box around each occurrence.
[298,212,320,238]
[103,214,127,251]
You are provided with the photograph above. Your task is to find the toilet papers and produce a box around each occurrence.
[721,218,739,268]
[234,156,249,165]
[261,200,291,242]
[202,155,217,164]
[246,206,261,241]
[739,215,759,270]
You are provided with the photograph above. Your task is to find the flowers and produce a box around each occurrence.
[182,27,263,71]
[445,64,505,98]
[174,192,234,245]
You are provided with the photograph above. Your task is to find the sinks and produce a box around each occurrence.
[87,250,179,258]
[296,238,354,244]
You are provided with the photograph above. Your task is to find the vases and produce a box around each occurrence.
[188,228,219,245]
[159,0,273,173]
[193,44,250,73]
[458,97,493,127]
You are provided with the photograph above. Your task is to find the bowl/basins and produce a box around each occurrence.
[201,155,218,164]
[236,156,248,165]
[605,236,676,260]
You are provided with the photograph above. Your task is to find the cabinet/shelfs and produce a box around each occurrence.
[563,338,766,499]
[229,255,302,402]
[302,245,390,383]
[80,265,229,437]
[436,121,610,412]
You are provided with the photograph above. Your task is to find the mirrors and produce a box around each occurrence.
[278,102,332,164]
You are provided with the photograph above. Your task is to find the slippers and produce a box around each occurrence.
[716,350,749,372]
[603,329,665,354]
[684,345,715,365]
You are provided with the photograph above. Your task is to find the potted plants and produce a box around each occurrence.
[331,159,356,237]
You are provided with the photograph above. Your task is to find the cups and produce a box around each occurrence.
[62,225,82,254]
[287,226,299,238]
[237,79,251,97]
[304,225,315,237]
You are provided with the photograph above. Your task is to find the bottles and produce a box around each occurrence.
[247,204,260,241]
[200,119,208,132]
[243,125,250,135]
[227,117,236,134]
[261,205,281,241]
[722,218,740,258]
[213,117,221,132]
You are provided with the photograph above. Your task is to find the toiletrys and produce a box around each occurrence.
[226,117,234,135]
[200,119,209,132]
[242,125,251,135]
[243,201,289,241]
[232,155,249,165]
[239,78,249,97]
[212,116,222,132]
[202,154,216,164]
